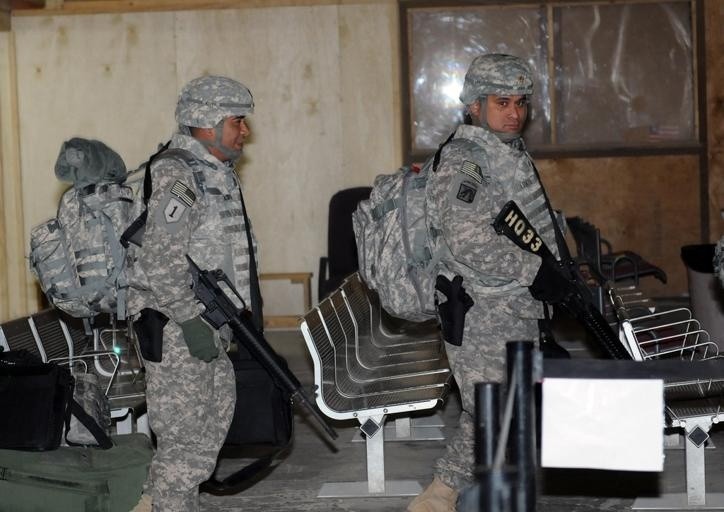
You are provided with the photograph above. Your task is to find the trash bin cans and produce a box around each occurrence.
[680,243,724,353]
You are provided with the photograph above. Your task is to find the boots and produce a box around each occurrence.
[406,473,461,512]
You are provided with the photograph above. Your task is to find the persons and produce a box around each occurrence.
[126,75,260,512]
[405,53,574,512]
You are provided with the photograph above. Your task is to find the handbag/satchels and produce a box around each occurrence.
[0,344,113,452]
[199,350,294,496]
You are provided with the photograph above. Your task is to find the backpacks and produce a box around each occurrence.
[352,139,491,322]
[30,148,204,320]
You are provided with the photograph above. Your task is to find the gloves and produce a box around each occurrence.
[528,263,573,305]
[182,316,218,362]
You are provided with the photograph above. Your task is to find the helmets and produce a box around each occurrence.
[175,75,255,128]
[459,54,533,105]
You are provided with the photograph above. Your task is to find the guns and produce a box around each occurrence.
[494,200,672,426]
[119,208,341,453]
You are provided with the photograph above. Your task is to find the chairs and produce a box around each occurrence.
[1,308,146,433]
